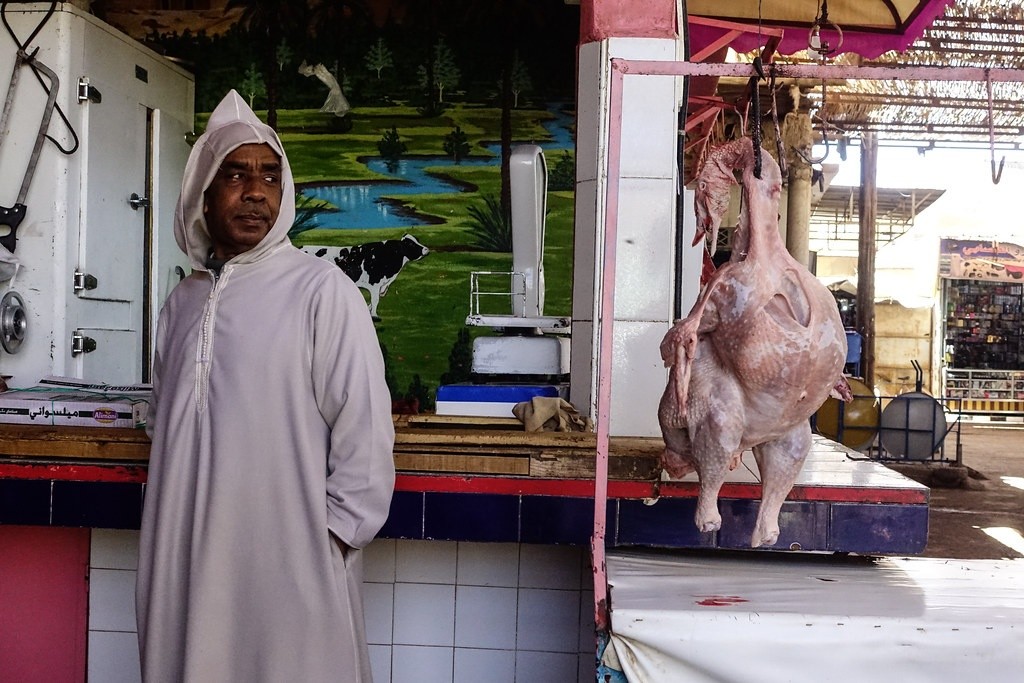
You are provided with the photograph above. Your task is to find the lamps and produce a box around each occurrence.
[808,0,823,61]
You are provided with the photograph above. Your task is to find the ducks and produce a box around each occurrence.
[656,136,852,548]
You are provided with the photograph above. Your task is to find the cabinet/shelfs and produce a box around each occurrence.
[0,3,196,392]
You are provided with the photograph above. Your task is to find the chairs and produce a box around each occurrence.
[843,330,862,379]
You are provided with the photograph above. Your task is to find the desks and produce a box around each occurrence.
[604,547,1024,682]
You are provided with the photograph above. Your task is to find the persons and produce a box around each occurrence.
[133,92,397,683]
